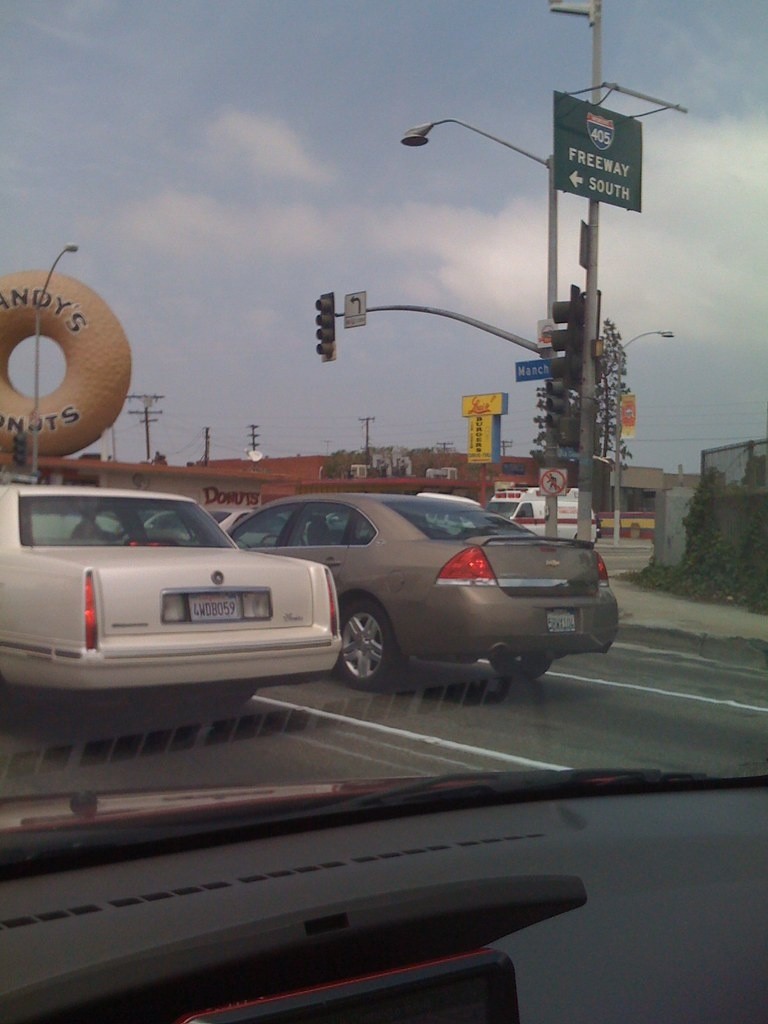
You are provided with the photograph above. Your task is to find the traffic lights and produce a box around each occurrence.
[314,291,336,363]
[544,379,574,448]
[13,433,27,465]
[549,283,587,387]
[566,457,579,490]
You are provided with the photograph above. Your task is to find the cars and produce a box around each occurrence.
[0,485,344,698]
[141,505,308,549]
[222,491,619,692]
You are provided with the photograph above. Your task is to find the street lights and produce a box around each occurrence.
[551,0,607,543]
[31,241,80,485]
[611,329,676,546]
[401,119,561,540]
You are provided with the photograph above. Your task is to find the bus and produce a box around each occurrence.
[597,509,655,540]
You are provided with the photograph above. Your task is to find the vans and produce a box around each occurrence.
[414,490,482,536]
[485,486,600,550]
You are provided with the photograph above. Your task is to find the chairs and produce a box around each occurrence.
[300,515,331,545]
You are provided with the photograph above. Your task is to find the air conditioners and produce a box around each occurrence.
[351,464,367,478]
[442,467,458,480]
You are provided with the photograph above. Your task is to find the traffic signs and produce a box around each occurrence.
[549,88,644,215]
[342,290,367,329]
